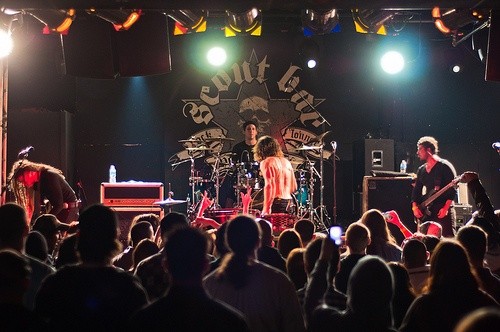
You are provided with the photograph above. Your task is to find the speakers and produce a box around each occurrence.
[112,207,165,252]
[365,139,394,175]
[362,175,420,234]
[454,205,472,231]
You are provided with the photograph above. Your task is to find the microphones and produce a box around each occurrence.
[19,147,34,155]
[492,142,500,150]
[247,152,250,162]
[331,141,336,150]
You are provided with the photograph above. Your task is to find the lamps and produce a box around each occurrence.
[0,6,149,40]
[302,7,343,35]
[431,5,491,37]
[350,8,400,35]
[160,8,210,36]
[224,6,266,39]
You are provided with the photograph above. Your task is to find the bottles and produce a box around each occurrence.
[400,159,407,173]
[109,165,116,183]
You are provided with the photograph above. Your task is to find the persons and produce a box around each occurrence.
[251,135,297,216]
[10,159,79,238]
[411,136,459,238]
[225,120,258,207]
[0,172,500,332]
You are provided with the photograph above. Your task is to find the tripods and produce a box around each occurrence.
[299,151,330,234]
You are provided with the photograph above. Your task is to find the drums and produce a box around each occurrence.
[294,170,310,181]
[195,179,216,199]
[296,185,308,208]
[249,188,299,237]
[235,161,261,191]
[189,177,202,206]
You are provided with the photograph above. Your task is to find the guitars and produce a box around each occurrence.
[413,171,480,224]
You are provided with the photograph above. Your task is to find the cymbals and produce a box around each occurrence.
[178,139,212,143]
[152,198,187,206]
[205,136,234,140]
[185,147,214,151]
[290,146,322,151]
